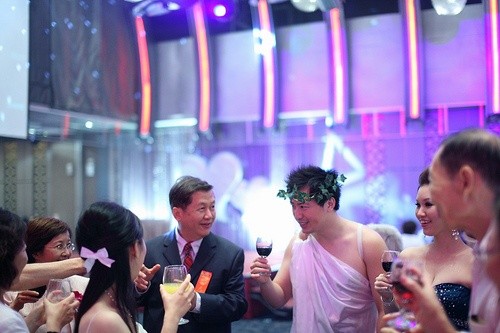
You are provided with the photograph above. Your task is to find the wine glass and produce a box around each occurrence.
[387,256,425,329]
[381,250,397,293]
[256,236,272,276]
[163,264,189,325]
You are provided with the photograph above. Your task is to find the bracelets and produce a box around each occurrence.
[380,295,395,306]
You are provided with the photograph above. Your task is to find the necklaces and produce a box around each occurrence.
[105,289,134,325]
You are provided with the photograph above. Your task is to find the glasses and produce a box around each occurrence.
[44,244,76,252]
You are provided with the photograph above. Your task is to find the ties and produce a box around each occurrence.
[182,244,194,272]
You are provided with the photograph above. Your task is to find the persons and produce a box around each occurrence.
[428,128,500,333]
[71,200,196,333]
[0,207,78,333]
[249,163,392,333]
[360,220,434,333]
[3,257,87,292]
[123,175,248,333]
[3,216,80,333]
[374,169,476,333]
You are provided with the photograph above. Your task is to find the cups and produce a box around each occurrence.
[47,279,72,304]
